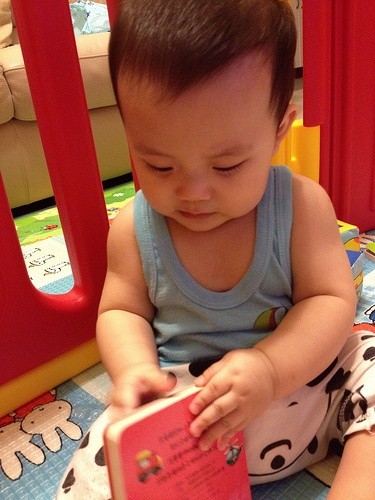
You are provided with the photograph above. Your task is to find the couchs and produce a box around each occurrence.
[0,0,133,210]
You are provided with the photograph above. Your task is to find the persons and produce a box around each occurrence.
[53,0,375,500]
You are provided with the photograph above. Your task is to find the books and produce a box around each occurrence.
[103,383,252,500]
